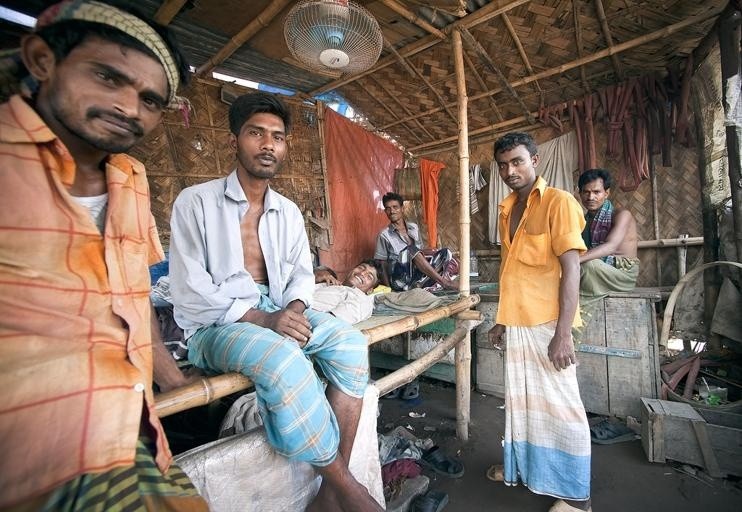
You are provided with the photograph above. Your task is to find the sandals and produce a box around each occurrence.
[382,379,419,399]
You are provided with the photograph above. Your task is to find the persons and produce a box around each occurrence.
[169,90,384,512]
[486,134,592,512]
[374,194,462,294]
[576,169,640,294]
[309,256,383,326]
[2,0,185,512]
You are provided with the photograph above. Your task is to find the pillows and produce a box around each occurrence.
[368,284,391,301]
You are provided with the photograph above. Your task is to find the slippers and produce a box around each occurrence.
[486,463,505,483]
[416,444,466,479]
[589,417,636,445]
[548,498,593,512]
[407,487,451,512]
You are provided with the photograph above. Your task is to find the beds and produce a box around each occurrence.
[476,286,662,425]
[369,281,501,423]
[147,285,483,445]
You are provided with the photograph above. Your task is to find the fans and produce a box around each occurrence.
[282,1,384,75]
